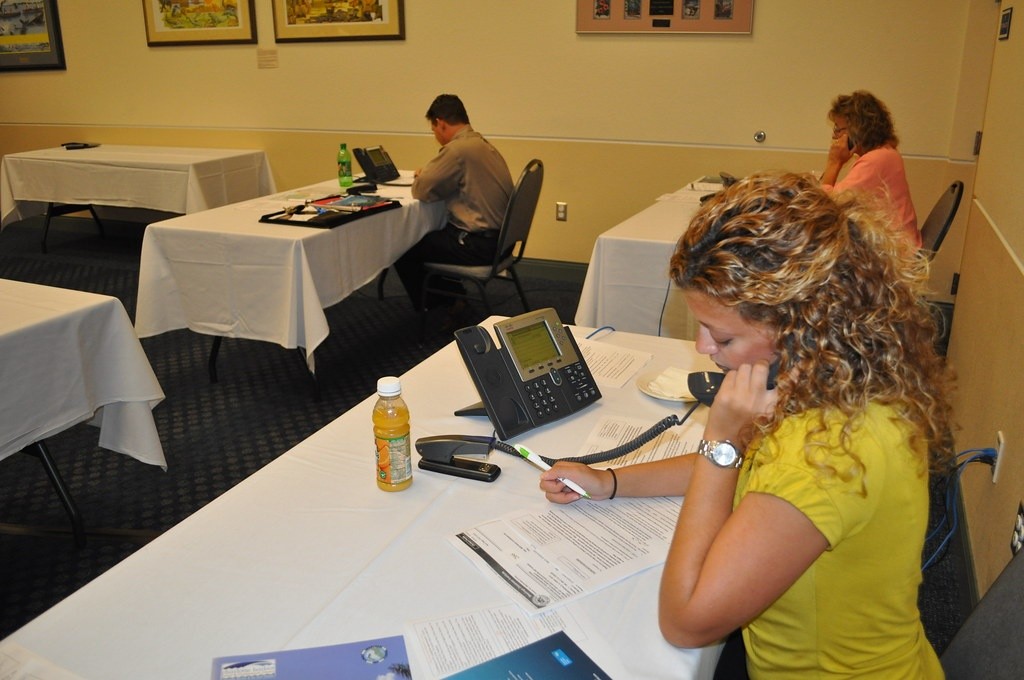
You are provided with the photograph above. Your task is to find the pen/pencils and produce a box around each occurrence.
[514,443,593,500]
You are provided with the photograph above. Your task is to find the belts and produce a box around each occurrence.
[475,231,499,240]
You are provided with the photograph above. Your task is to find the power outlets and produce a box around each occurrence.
[555,202,567,221]
[988,432,1003,484]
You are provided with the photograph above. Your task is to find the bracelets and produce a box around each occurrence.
[607,467,618,500]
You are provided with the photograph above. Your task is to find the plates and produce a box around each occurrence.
[635,367,697,403]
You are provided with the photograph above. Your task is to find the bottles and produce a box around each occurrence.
[372,376,413,491]
[336,143,352,187]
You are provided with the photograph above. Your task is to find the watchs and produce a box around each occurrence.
[697,439,747,470]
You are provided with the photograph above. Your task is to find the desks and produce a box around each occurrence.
[0,310,727,680]
[0,280,168,525]
[0,146,277,257]
[574,176,756,354]
[133,168,449,389]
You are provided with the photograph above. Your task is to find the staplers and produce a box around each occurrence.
[346,184,378,196]
[415,434,501,483]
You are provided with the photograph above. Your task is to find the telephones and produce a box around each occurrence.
[848,137,857,150]
[352,144,401,183]
[688,340,819,408]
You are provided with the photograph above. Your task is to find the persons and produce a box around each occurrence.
[540,170,945,680]
[390,93,515,341]
[817,90,923,259]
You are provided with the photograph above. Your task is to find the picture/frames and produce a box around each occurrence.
[0,0,66,71]
[271,0,405,44]
[142,0,258,47]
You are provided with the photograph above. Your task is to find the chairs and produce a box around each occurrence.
[422,157,544,315]
[939,547,1024,680]
[917,179,965,265]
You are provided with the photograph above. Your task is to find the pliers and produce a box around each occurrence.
[60,142,101,149]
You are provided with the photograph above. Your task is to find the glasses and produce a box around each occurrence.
[833,122,852,134]
